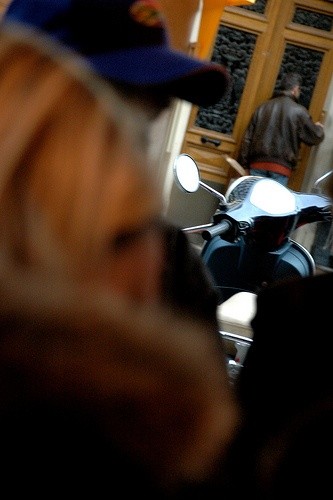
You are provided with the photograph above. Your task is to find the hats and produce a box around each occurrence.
[2,0,233,110]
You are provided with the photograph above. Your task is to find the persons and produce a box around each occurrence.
[0,0,246,499]
[237,72,327,188]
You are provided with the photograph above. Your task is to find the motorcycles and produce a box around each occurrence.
[172,147,333,389]
